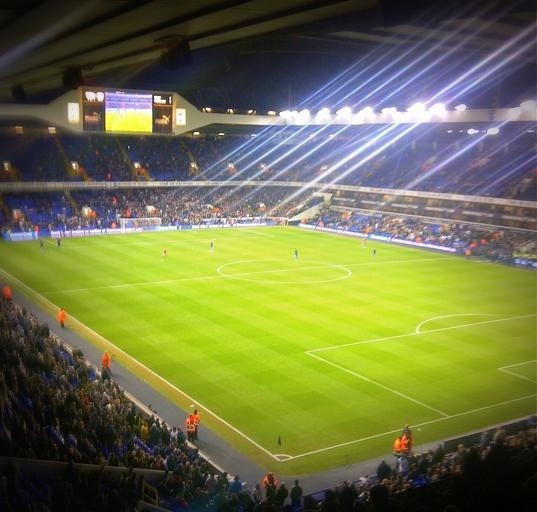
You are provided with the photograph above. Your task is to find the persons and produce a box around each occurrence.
[0,129,536,269]
[253,413,537,511]
[1,285,253,512]
